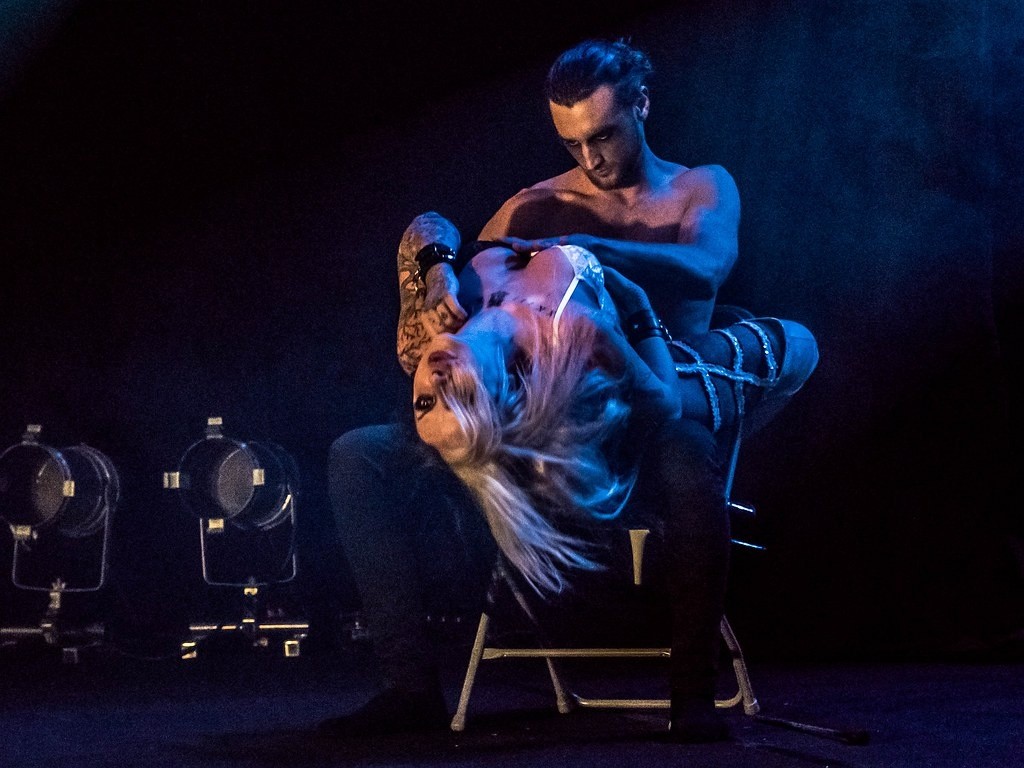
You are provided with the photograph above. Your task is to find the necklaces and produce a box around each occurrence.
[468,291,556,319]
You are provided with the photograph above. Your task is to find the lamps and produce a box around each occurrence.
[163,416,299,619]
[0,424,120,592]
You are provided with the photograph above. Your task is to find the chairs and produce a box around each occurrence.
[450,416,745,731]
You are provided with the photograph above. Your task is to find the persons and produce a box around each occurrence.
[316,40,741,742]
[396,208,821,615]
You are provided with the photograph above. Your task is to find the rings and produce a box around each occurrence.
[440,311,448,320]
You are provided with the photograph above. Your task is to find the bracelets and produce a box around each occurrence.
[624,310,658,327]
[629,319,663,334]
[627,329,673,345]
[414,242,457,283]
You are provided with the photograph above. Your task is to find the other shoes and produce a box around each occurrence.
[666,664,724,744]
[321,690,447,739]
